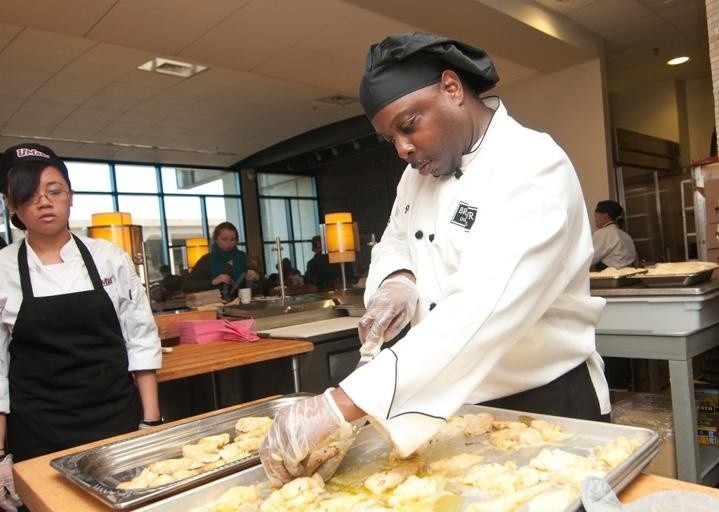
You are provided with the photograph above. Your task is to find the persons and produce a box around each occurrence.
[0,143,166,511]
[258,29,614,489]
[591,199,641,394]
[159,220,361,304]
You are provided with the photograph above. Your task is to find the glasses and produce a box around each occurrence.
[20,188,71,204]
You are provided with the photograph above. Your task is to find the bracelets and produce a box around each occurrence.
[138,417,167,429]
[0,448,7,460]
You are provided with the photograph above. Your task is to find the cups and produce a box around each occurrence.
[238,288,253,304]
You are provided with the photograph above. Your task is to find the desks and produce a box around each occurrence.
[129,336,315,412]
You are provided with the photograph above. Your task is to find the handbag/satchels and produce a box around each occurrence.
[217,272,247,303]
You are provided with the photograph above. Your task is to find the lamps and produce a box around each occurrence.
[88,213,151,314]
[185,237,210,275]
[319,213,360,291]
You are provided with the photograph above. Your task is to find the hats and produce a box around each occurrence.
[359,32,502,125]
[2,143,68,195]
[595,200,623,218]
